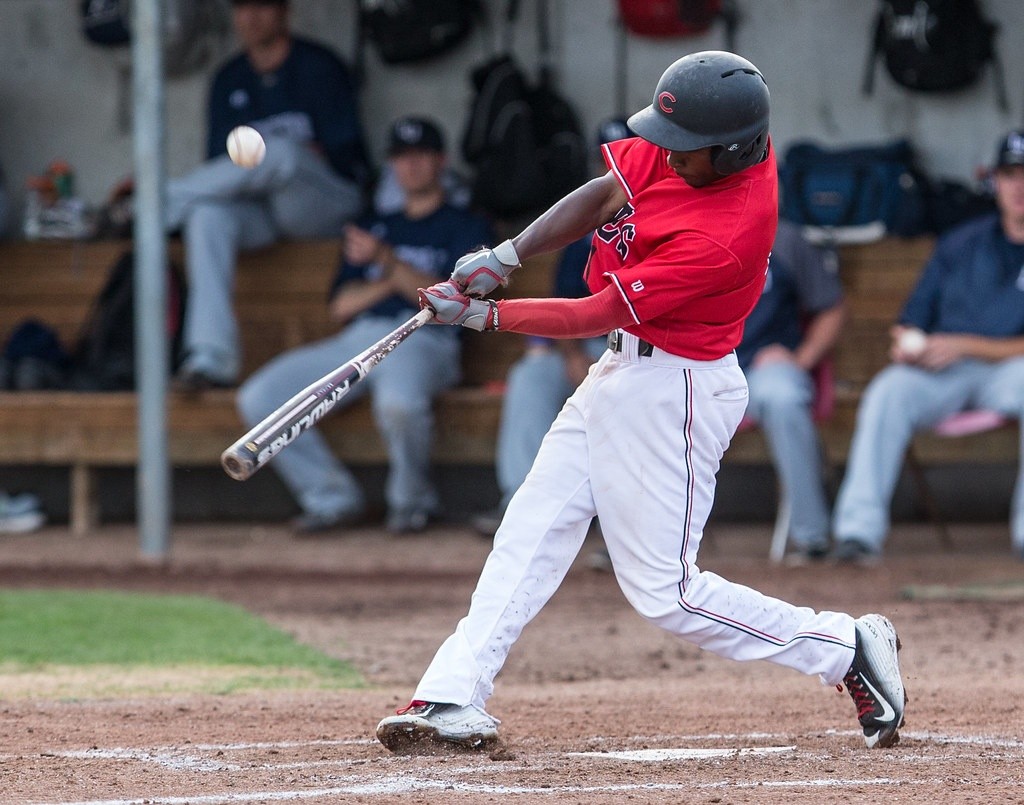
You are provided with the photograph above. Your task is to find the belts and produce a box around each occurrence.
[606,328,655,356]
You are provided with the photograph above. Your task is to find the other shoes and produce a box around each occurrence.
[783,545,827,577]
[0,493,47,538]
[828,541,878,578]
[77,206,131,243]
[385,508,432,536]
[186,370,236,395]
[292,510,363,535]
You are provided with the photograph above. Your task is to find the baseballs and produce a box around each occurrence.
[226,126,266,167]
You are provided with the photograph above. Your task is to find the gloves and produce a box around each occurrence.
[417,282,490,332]
[447,239,520,298]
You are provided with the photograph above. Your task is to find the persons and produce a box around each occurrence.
[821,129,1024,559]
[476,114,638,536]
[234,113,484,536]
[735,225,847,560]
[377,51,909,753]
[163,0,379,387]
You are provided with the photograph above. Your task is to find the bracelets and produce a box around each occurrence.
[486,299,499,334]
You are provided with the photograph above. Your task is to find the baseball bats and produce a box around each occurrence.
[220,305,436,481]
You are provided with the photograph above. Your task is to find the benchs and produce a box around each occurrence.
[0,233,1024,539]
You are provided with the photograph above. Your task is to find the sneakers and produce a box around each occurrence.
[375,702,501,755]
[839,614,908,749]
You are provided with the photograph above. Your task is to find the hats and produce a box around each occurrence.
[993,129,1024,166]
[385,113,444,156]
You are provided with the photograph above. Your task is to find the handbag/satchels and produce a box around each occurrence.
[777,140,930,239]
[0,318,95,397]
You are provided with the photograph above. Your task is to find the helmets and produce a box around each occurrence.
[625,49,771,174]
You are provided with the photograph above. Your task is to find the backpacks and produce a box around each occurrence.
[880,0,993,96]
[615,0,717,42]
[363,0,479,73]
